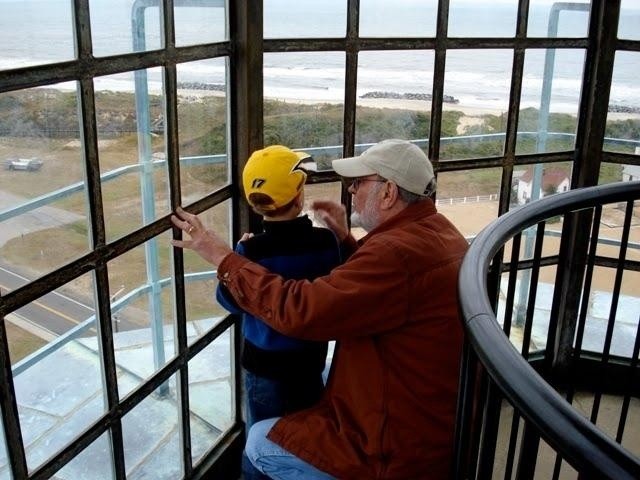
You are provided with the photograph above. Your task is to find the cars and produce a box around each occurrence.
[7,157,43,172]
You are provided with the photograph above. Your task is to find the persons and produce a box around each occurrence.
[216,143,343,421]
[170,138,468,480]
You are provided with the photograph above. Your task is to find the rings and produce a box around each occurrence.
[188,226,195,233]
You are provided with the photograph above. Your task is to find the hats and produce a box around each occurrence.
[332,139,434,196]
[243,145,316,211]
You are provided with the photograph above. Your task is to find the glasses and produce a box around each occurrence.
[353,178,385,190]
